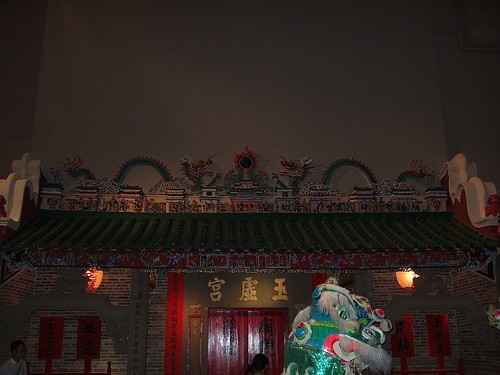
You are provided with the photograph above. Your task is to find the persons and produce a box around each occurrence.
[245,353,269,375]
[0,339,28,375]
